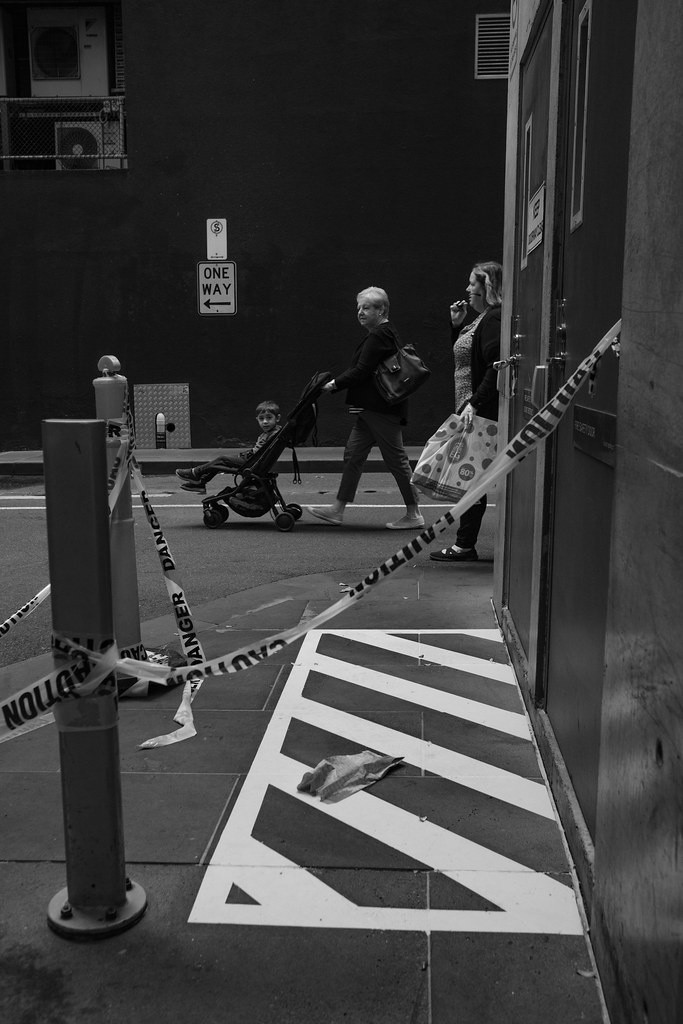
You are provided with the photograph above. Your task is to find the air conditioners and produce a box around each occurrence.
[28,6,109,100]
[56,122,128,170]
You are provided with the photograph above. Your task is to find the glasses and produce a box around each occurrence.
[456,292,481,310]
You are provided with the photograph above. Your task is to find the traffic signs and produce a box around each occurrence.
[197,261,237,317]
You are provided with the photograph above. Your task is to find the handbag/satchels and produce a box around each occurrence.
[409,411,499,505]
[371,327,432,403]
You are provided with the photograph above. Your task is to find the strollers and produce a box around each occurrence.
[202,371,334,533]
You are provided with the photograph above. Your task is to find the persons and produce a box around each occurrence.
[429,261,502,562]
[306,284,426,530]
[176,401,284,494]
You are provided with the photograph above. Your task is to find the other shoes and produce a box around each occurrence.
[386,515,426,531]
[308,506,342,524]
[430,546,479,562]
[180,483,205,492]
[176,469,201,483]
